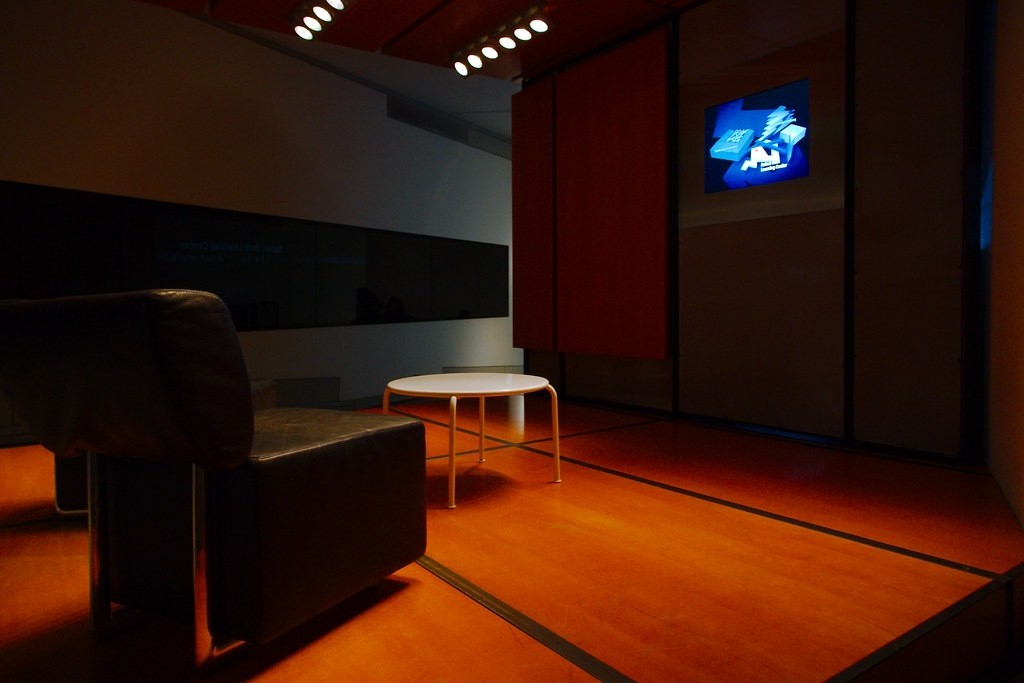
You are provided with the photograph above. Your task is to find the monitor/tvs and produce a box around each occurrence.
[703,77,810,194]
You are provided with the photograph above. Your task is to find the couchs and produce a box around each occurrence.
[0,289,426,672]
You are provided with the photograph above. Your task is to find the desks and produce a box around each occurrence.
[382,372,562,509]
